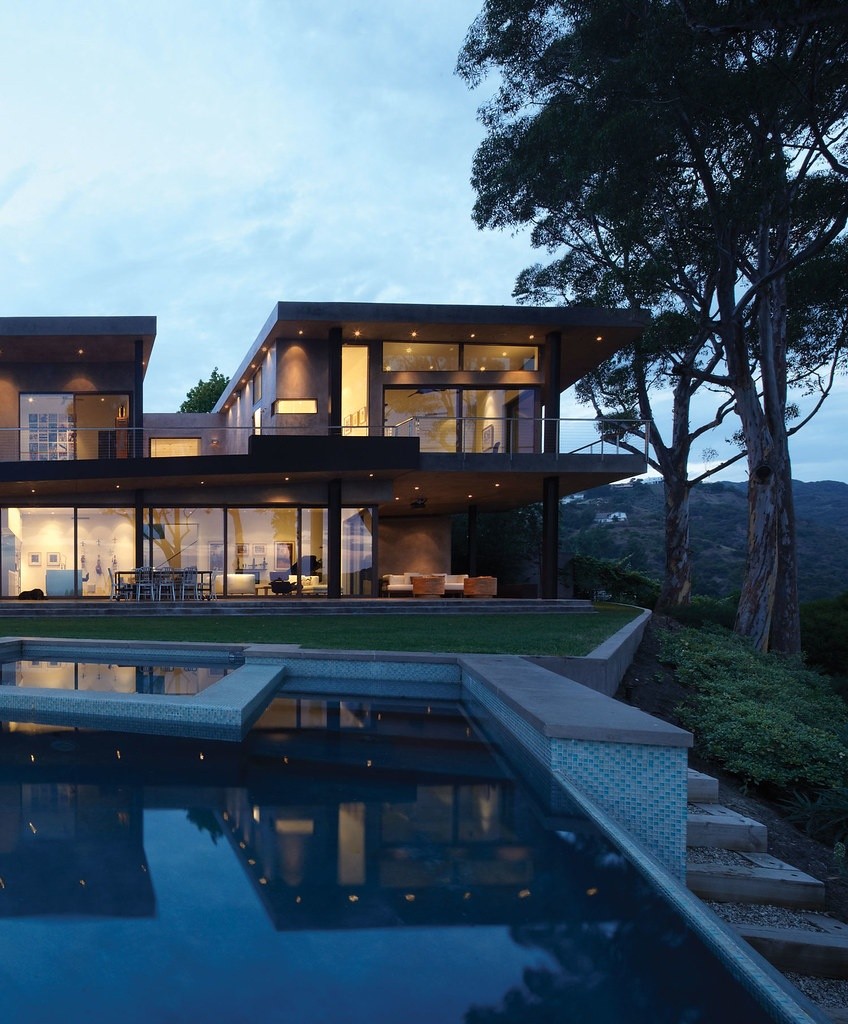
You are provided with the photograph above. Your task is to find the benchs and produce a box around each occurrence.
[412,577,445,598]
[381,575,469,598]
[303,585,344,595]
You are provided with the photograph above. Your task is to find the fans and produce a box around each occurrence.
[45,396,85,406]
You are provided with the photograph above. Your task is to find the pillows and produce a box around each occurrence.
[404,572,419,584]
[432,573,448,583]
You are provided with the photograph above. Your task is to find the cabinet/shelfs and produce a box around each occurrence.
[115,417,128,458]
[1,534,22,596]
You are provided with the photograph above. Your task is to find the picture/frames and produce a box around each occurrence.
[208,541,224,575]
[46,551,61,566]
[28,551,42,566]
[236,543,249,556]
[352,411,359,426]
[253,545,267,556]
[481,424,494,452]
[359,407,367,424]
[343,414,352,435]
[274,541,295,571]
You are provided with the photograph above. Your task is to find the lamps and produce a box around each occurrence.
[211,438,219,444]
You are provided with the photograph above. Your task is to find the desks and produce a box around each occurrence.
[464,578,497,598]
[114,570,213,602]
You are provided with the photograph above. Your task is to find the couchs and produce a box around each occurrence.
[288,575,319,594]
[215,574,256,595]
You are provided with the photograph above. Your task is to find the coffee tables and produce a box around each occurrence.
[255,585,271,595]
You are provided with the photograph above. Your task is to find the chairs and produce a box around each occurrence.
[270,581,291,595]
[493,442,500,453]
[107,566,199,601]
